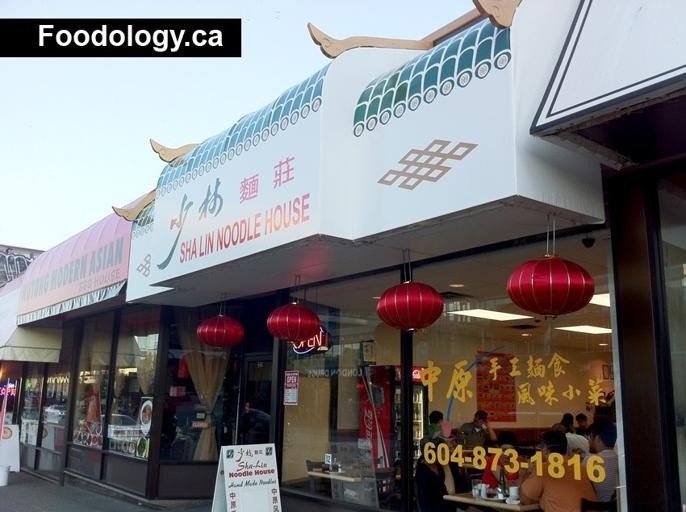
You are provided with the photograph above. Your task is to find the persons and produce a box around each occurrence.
[416,390,620,511]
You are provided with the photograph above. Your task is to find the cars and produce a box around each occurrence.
[239,409,270,443]
[45,405,67,417]
[101,414,142,441]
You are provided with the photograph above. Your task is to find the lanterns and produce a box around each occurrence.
[376,280,444,331]
[506,254,597,319]
[196,315,244,349]
[267,303,321,343]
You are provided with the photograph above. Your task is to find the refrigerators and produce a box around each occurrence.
[358,364,429,488]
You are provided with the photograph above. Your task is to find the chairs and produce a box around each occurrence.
[305,459,333,495]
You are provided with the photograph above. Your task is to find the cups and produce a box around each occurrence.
[509,487,520,501]
[471,479,487,500]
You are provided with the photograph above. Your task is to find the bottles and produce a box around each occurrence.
[496,469,506,498]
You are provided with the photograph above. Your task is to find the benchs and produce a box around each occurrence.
[450,426,603,452]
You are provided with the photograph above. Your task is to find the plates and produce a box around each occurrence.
[484,497,508,502]
